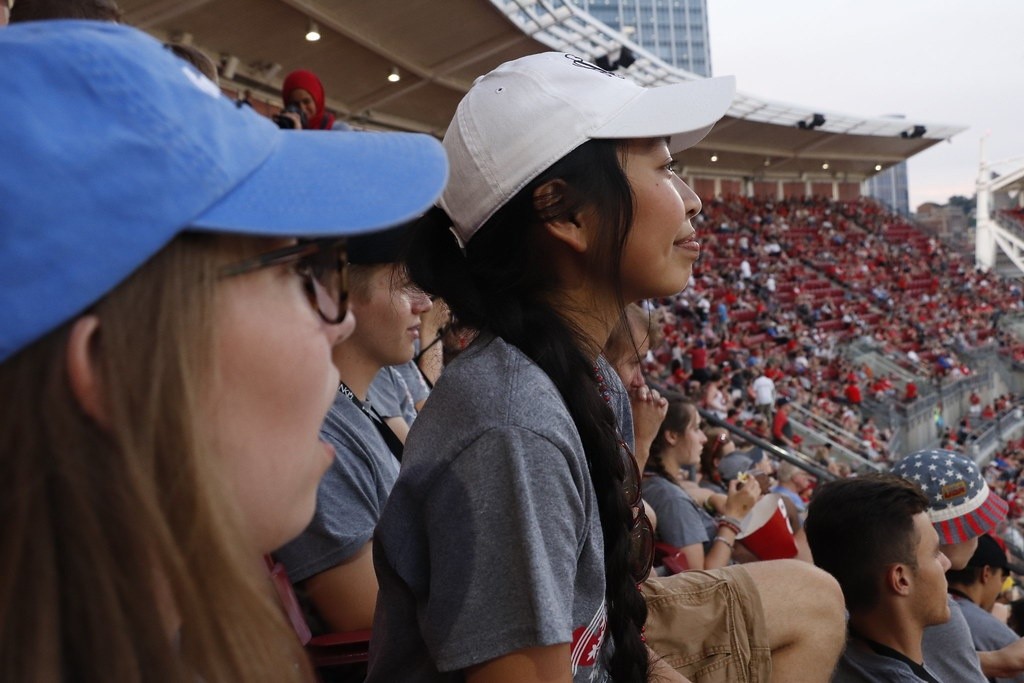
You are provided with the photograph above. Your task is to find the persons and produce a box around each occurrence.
[1,0,849,682]
[639,193,1024,683]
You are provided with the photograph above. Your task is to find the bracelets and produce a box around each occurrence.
[720,515,742,531]
[713,536,734,552]
[705,493,713,509]
[718,520,740,533]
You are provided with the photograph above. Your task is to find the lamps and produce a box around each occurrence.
[797,113,825,131]
[304,19,321,42]
[822,160,830,170]
[251,62,283,81]
[764,158,771,166]
[220,56,239,79]
[387,66,400,82]
[901,125,926,138]
[710,152,719,161]
[595,46,635,72]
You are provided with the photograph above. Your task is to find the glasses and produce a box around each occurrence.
[617,439,655,586]
[194,233,351,326]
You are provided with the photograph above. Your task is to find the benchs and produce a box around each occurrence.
[713,200,1024,375]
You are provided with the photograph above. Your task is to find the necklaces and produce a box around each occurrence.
[594,363,647,643]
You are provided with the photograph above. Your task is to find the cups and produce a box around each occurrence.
[733,493,799,561]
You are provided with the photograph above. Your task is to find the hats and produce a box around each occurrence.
[889,448,1010,545]
[0,19,449,362]
[438,51,735,247]
